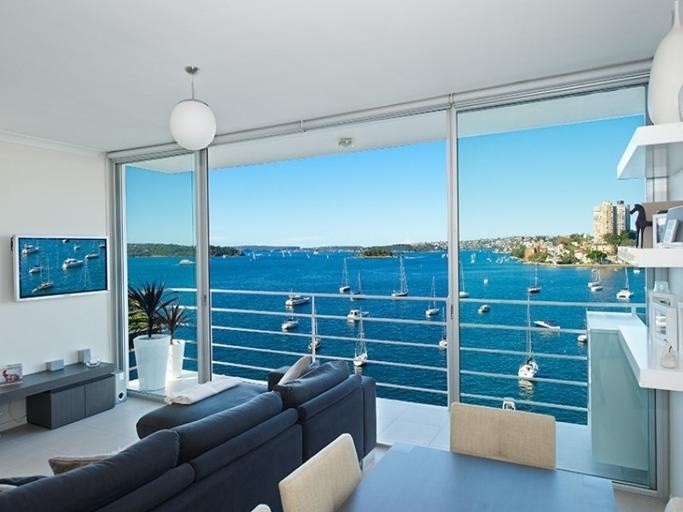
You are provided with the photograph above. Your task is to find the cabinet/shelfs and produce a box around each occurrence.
[616,121,683,395]
[0,359,116,430]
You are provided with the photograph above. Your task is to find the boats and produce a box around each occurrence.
[391,256,409,297]
[424,276,439,316]
[338,258,365,301]
[458,259,641,395]
[307,309,321,350]
[438,306,447,350]
[285,288,307,307]
[179,259,195,265]
[280,316,300,330]
[346,303,370,366]
[21,238,105,293]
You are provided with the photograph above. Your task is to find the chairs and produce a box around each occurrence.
[448,398,557,468]
[276,429,364,512]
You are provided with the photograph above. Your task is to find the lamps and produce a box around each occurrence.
[168,66,217,153]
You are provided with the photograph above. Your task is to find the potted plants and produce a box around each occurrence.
[126,278,186,391]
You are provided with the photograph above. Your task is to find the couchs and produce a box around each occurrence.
[0,354,380,512]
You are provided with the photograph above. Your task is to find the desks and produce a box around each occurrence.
[585,311,650,479]
[335,442,618,512]
